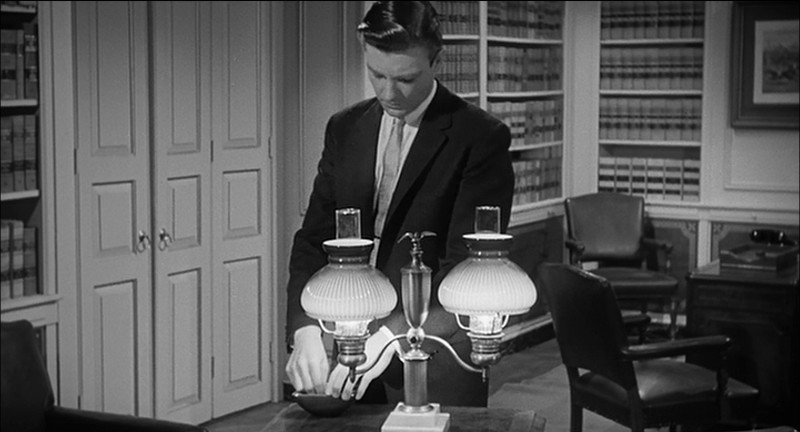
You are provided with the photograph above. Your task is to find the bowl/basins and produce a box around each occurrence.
[292,387,356,416]
[750,228,787,245]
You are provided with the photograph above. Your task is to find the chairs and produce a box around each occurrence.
[563,190,681,343]
[541,261,758,432]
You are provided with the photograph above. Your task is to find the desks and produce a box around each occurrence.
[685,254,800,377]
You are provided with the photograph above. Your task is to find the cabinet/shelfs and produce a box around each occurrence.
[423,0,482,108]
[0,0,60,407]
[484,1,569,228]
[596,0,706,207]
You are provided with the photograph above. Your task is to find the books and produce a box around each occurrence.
[512,148,563,205]
[488,0,563,40]
[489,100,563,146]
[0,220,37,298]
[430,0,480,35]
[487,44,562,92]
[601,0,704,40]
[598,153,699,203]
[601,44,702,92]
[600,98,701,141]
[0,30,38,100]
[0,115,36,192]
[434,45,478,93]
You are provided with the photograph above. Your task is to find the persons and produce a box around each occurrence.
[285,0,516,410]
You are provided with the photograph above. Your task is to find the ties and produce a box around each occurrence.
[366,117,406,267]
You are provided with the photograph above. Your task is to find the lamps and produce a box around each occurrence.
[301,207,537,432]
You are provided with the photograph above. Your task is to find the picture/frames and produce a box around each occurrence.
[729,1,800,131]
